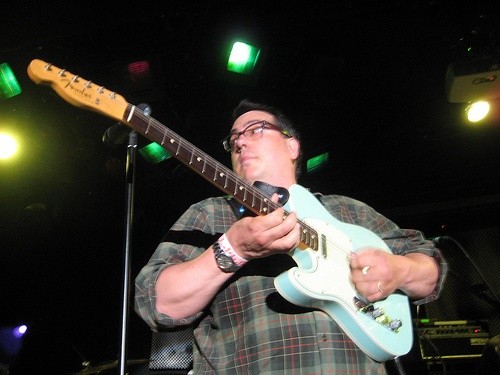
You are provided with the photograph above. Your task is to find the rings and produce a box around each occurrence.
[378,282,384,293]
[361,266,369,275]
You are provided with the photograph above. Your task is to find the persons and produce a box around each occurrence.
[137,102,448,375]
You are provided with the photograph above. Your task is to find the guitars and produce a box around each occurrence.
[26,59,416,363]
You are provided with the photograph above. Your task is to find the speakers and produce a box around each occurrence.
[396,202,500,375]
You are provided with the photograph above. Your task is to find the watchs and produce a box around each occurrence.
[212,241,242,273]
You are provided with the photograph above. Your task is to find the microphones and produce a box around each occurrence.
[102,103,152,147]
[425,236,450,243]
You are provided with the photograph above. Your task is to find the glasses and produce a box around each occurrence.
[223,120,292,154]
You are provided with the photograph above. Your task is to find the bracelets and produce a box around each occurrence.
[217,233,249,269]
